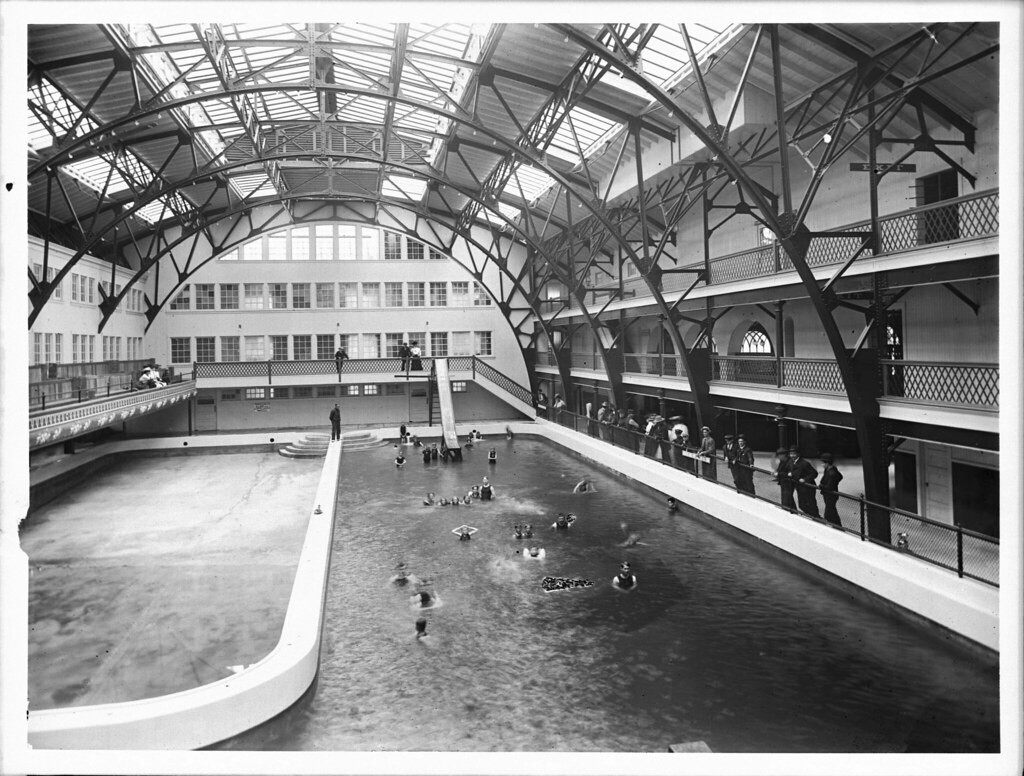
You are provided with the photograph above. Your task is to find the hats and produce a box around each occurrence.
[554,393,562,399]
[786,444,802,453]
[735,434,746,440]
[411,341,418,345]
[724,435,735,440]
[338,347,344,351]
[652,415,666,423]
[775,447,788,455]
[601,401,609,408]
[334,402,341,408]
[673,429,683,433]
[700,426,713,433]
[144,366,152,372]
[820,452,834,463]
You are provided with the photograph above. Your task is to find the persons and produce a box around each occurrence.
[335,348,349,373]
[523,546,546,560]
[553,393,565,421]
[394,452,406,468]
[512,523,535,540]
[329,403,341,443]
[468,431,482,439]
[539,389,547,418]
[423,443,444,463]
[452,524,478,540]
[487,447,497,464]
[613,562,636,583]
[412,434,424,447]
[666,496,675,509]
[506,427,513,440]
[584,402,688,467]
[773,444,844,526]
[424,476,495,506]
[141,365,170,389]
[399,423,407,440]
[549,513,571,530]
[571,474,594,494]
[696,426,755,494]
[412,617,427,639]
[398,342,423,371]
